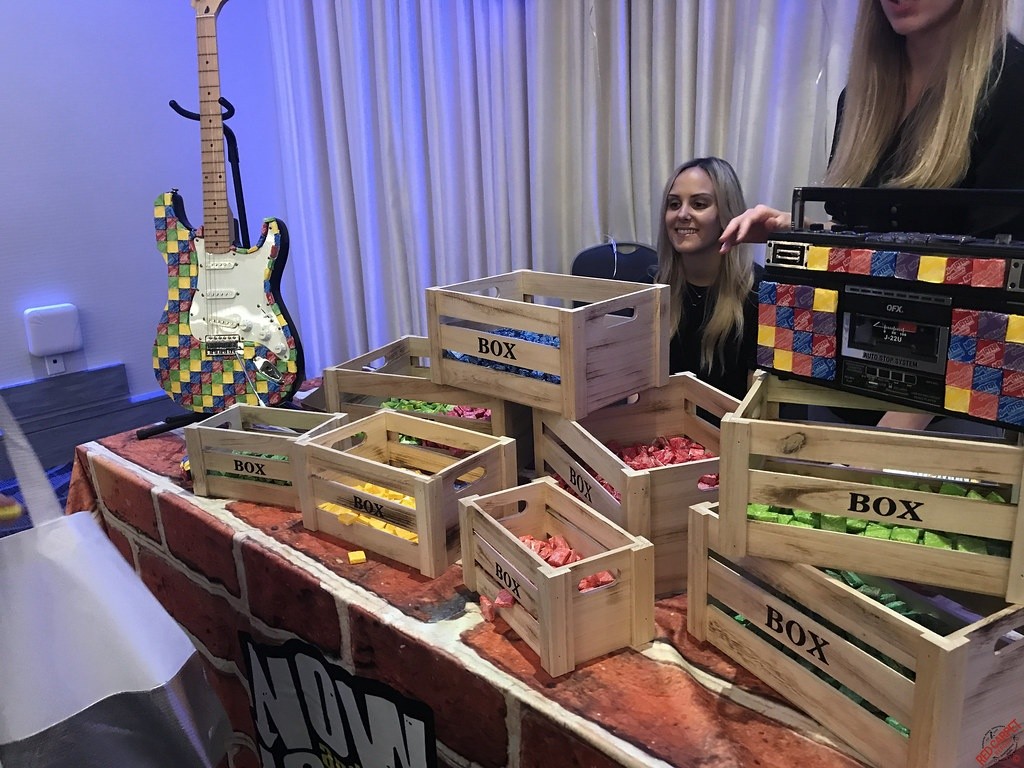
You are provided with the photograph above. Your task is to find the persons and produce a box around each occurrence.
[625,157,808,428]
[719,0,1024,444]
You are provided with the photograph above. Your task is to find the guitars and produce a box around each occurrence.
[151,0,306,415]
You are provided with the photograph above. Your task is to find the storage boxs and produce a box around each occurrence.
[684,501,1024,768]
[755,185,1024,434]
[424,268,672,419]
[457,476,659,678]
[321,332,536,472]
[529,370,741,599]
[186,405,352,511]
[718,367,1024,607]
[292,407,522,582]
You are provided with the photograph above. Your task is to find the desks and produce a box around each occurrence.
[72,377,1024,768]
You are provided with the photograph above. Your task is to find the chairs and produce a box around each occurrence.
[567,241,657,319]
